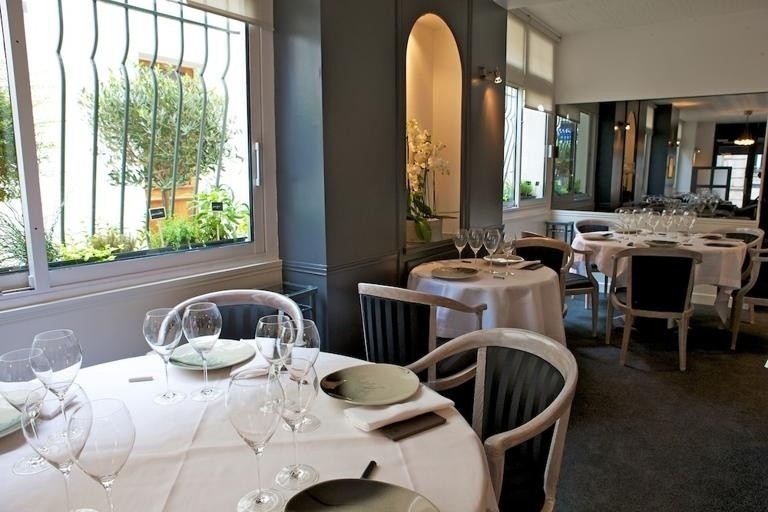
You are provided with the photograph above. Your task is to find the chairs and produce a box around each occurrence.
[399,322,583,511]
[155,287,306,356]
[616,194,758,217]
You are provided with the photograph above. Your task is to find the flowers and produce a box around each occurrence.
[623,155,635,190]
[406,120,456,243]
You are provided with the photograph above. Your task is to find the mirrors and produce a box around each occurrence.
[549,92,768,219]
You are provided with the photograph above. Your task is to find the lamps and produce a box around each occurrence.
[732,109,757,146]
[614,120,631,132]
[478,65,503,84]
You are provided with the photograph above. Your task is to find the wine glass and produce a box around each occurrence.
[276,319,322,432]
[254,314,295,413]
[63,398,136,512]
[499,231,516,277]
[181,301,227,403]
[0,347,54,476]
[452,228,467,268]
[614,208,698,243]
[483,229,500,275]
[268,358,319,490]
[467,227,483,271]
[646,189,720,218]
[28,327,85,444]
[141,307,187,407]
[20,382,96,512]
[226,369,288,512]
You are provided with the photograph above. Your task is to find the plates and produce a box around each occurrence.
[646,240,676,248]
[482,253,523,264]
[431,265,479,280]
[282,479,438,512]
[167,339,255,370]
[1,408,40,439]
[319,362,421,408]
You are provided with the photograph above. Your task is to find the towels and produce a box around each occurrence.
[227,337,278,383]
[0,380,75,421]
[343,384,455,432]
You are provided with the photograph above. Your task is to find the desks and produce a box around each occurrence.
[0,339,504,512]
